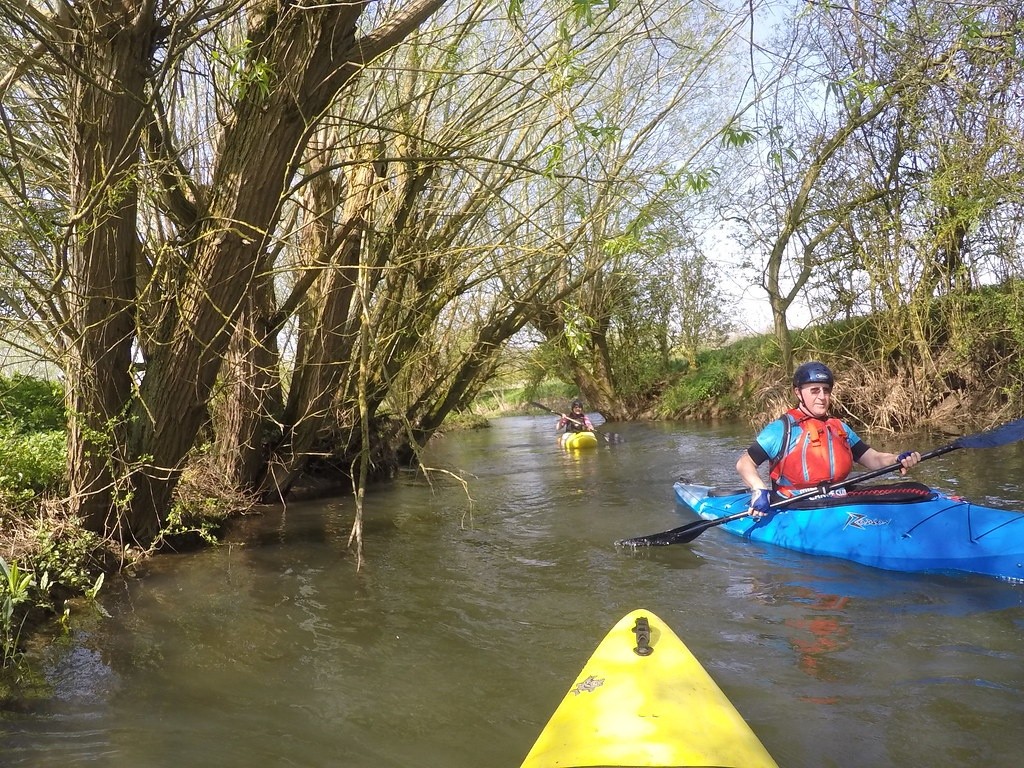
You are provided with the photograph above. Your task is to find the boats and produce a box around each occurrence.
[672,477,1024,584]
[520,609,780,768]
[557,431,598,448]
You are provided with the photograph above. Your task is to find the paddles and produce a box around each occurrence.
[527,399,627,445]
[618,415,1024,548]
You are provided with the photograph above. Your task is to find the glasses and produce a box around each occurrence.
[810,388,831,394]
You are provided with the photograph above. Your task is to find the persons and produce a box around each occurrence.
[735,362,921,517]
[556,400,596,436]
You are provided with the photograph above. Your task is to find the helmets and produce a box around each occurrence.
[571,399,583,410]
[793,362,833,388]
